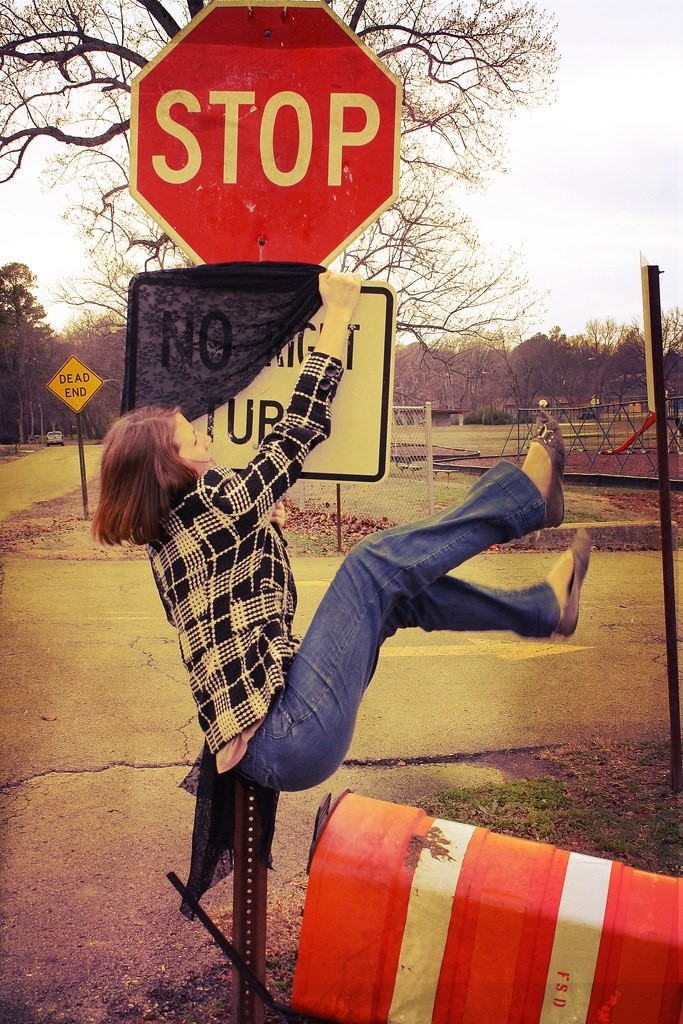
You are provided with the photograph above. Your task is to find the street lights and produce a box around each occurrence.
[588,358,597,365]
[482,372,493,425]
[397,387,404,406]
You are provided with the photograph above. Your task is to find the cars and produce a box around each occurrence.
[579,412,596,420]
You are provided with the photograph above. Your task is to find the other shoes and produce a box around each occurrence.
[554,528,590,638]
[532,409,565,528]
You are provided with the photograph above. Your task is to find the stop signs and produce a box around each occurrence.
[131,0,400,273]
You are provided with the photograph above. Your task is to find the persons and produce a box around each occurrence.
[92,270,590,792]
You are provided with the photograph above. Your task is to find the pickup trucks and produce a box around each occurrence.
[45,432,63,446]
